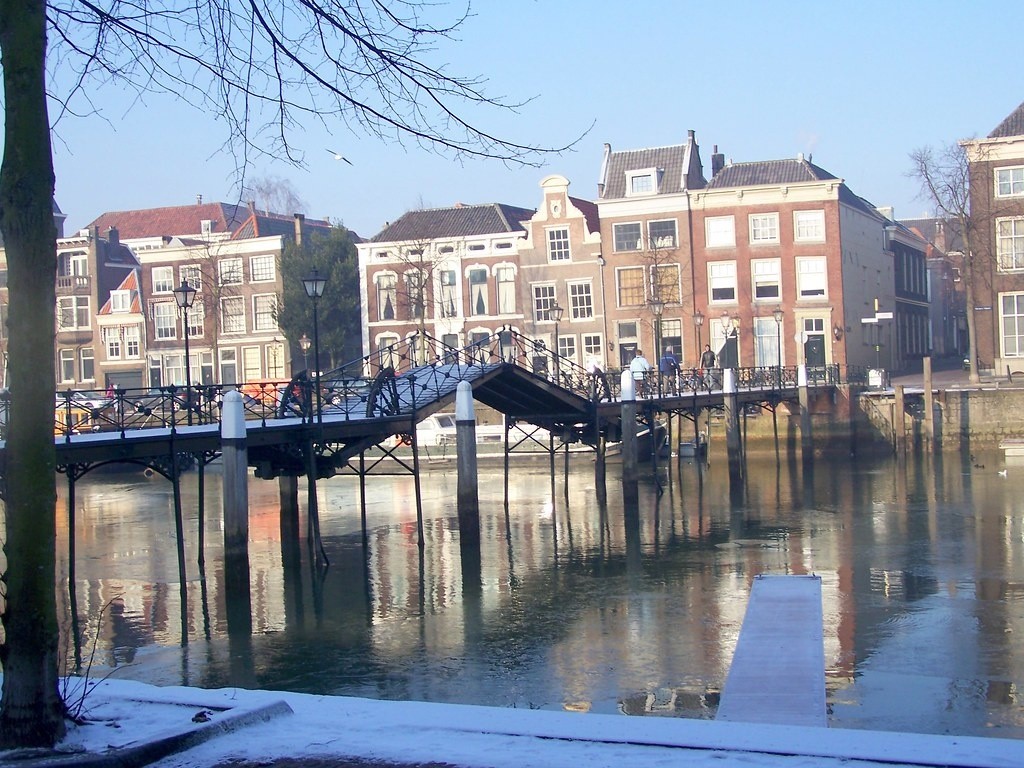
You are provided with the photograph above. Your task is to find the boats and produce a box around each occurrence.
[678,433,706,458]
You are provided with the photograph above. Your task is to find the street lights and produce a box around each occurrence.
[297,333,312,381]
[299,264,322,424]
[719,310,731,368]
[771,304,784,389]
[547,301,564,387]
[171,276,198,426]
[649,300,665,399]
[730,313,742,367]
[692,308,705,391]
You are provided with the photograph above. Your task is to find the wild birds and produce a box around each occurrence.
[324,148,355,167]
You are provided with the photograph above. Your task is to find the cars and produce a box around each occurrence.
[131,385,201,413]
[962,349,970,370]
[323,377,369,406]
[55,388,115,410]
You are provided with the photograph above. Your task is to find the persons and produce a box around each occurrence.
[700,344,715,390]
[630,349,650,397]
[107,383,116,396]
[659,346,680,396]
